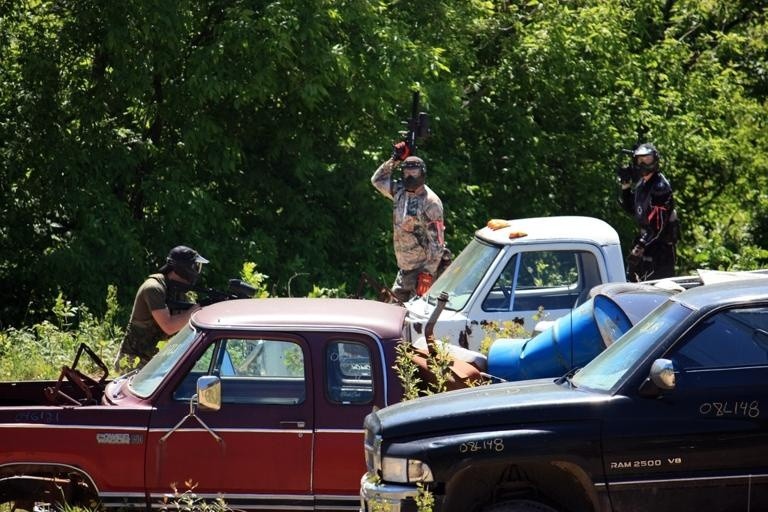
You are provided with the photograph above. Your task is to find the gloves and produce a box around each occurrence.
[416,271,432,296]
[625,243,645,266]
[392,140,409,162]
[618,163,634,184]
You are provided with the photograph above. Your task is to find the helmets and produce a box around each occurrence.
[634,142,659,173]
[401,156,427,188]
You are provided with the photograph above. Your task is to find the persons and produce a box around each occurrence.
[371,142,446,303]
[113,246,209,376]
[619,143,678,282]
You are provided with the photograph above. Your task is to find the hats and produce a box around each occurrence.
[166,245,211,267]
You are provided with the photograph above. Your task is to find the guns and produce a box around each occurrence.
[395,91,428,161]
[165,279,256,311]
[618,117,648,185]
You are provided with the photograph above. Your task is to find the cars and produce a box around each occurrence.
[0,285,479,512]
